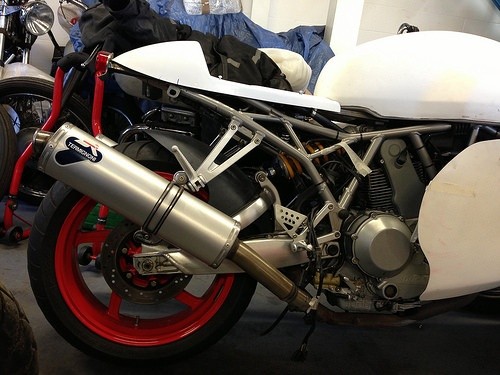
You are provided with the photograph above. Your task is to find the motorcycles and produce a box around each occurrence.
[0,0,500,375]
